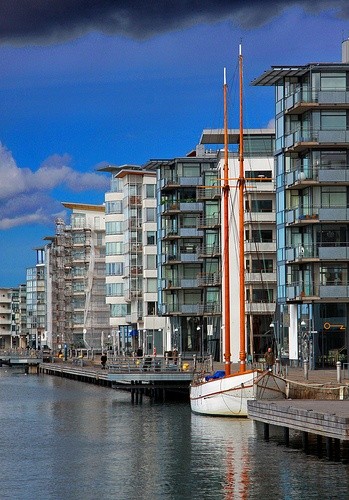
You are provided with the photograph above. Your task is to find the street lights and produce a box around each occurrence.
[196,321,203,362]
[301,319,310,381]
[269,322,275,351]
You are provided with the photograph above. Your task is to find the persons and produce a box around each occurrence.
[58,350,63,359]
[100,353,108,370]
[144,355,152,371]
[137,346,143,359]
[264,347,276,374]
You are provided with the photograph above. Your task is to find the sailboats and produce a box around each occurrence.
[189,42,286,417]
